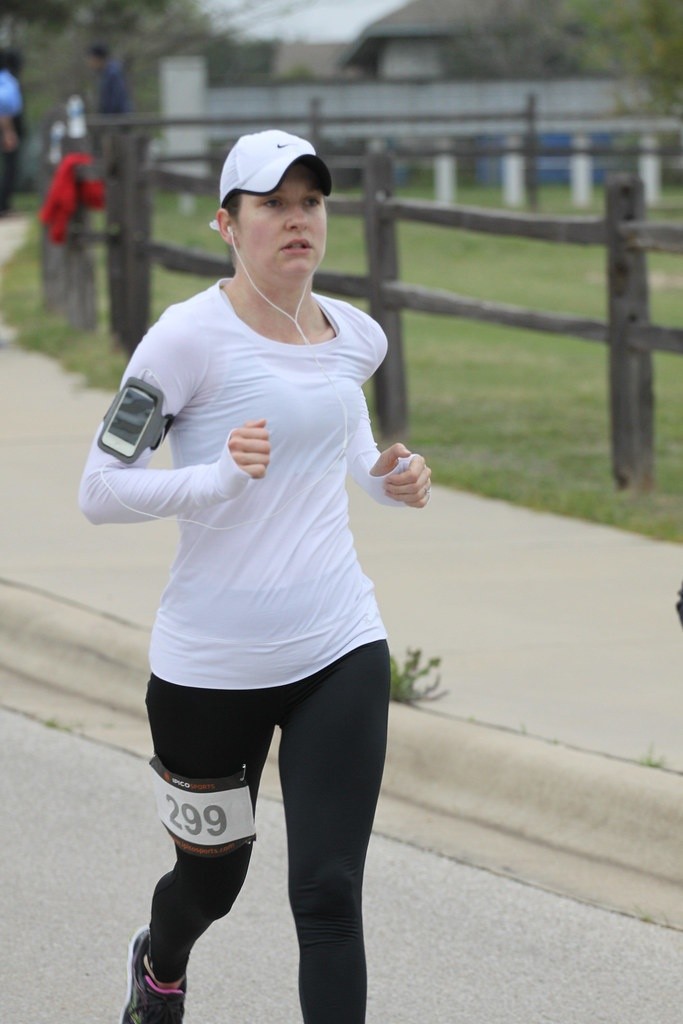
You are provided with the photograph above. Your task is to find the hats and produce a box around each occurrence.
[219,128,330,208]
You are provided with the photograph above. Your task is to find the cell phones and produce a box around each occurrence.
[101,386,157,457]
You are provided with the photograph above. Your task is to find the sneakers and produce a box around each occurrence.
[122,923,189,1024]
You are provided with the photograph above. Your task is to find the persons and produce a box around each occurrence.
[87,41,132,115]
[81,129,432,1024]
[0,47,22,218]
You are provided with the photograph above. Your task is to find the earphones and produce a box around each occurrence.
[227,225,233,237]
